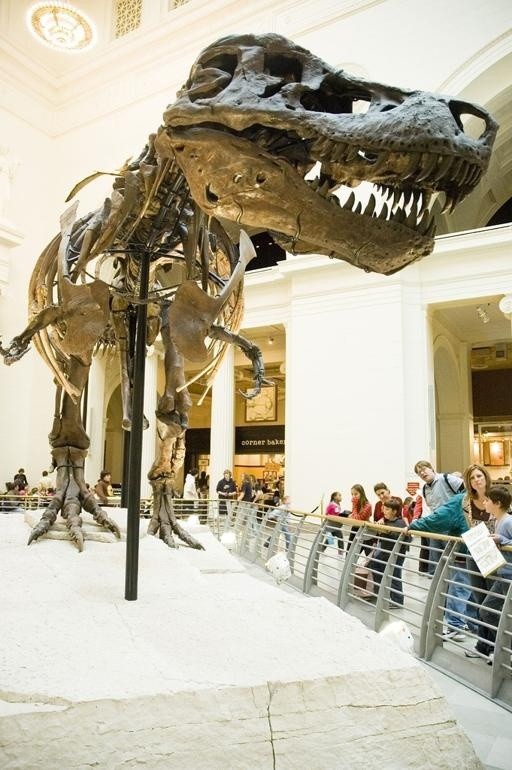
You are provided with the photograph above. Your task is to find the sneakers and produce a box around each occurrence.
[442,626,497,667]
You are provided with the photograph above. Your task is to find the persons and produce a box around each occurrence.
[321,461,512,667]
[182,469,292,552]
[4,468,114,511]
